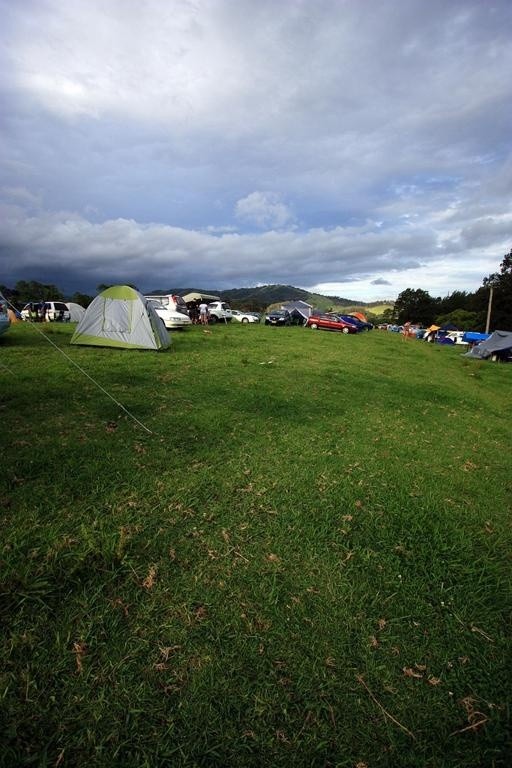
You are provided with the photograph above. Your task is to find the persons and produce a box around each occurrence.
[25,298,48,321]
[400,320,413,342]
[188,297,209,324]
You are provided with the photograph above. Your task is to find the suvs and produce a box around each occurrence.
[207,301,232,325]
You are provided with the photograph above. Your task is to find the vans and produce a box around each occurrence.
[143,294,188,314]
[20,301,71,321]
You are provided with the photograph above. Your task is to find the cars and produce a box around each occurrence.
[265,309,291,326]
[145,298,193,331]
[231,309,261,324]
[307,313,373,334]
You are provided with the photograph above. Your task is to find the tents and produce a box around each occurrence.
[282,300,312,319]
[349,311,368,323]
[64,302,86,322]
[68,283,173,352]
